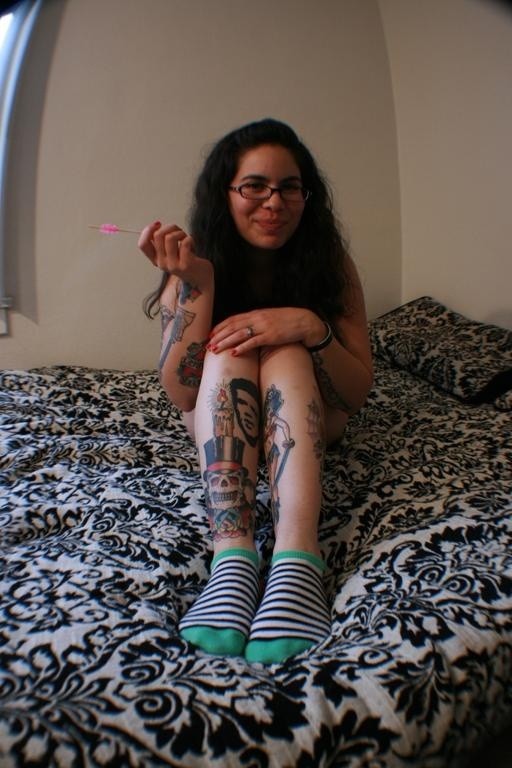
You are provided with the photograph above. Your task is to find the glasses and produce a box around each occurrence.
[228,182,311,202]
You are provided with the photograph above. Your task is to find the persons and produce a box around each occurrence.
[136,116,378,668]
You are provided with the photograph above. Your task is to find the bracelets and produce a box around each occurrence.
[308,321,333,351]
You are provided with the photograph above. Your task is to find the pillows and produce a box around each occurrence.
[367,295,512,411]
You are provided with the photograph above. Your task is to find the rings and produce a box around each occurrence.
[247,326,254,338]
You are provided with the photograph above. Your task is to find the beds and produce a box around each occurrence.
[0,352,512,768]
